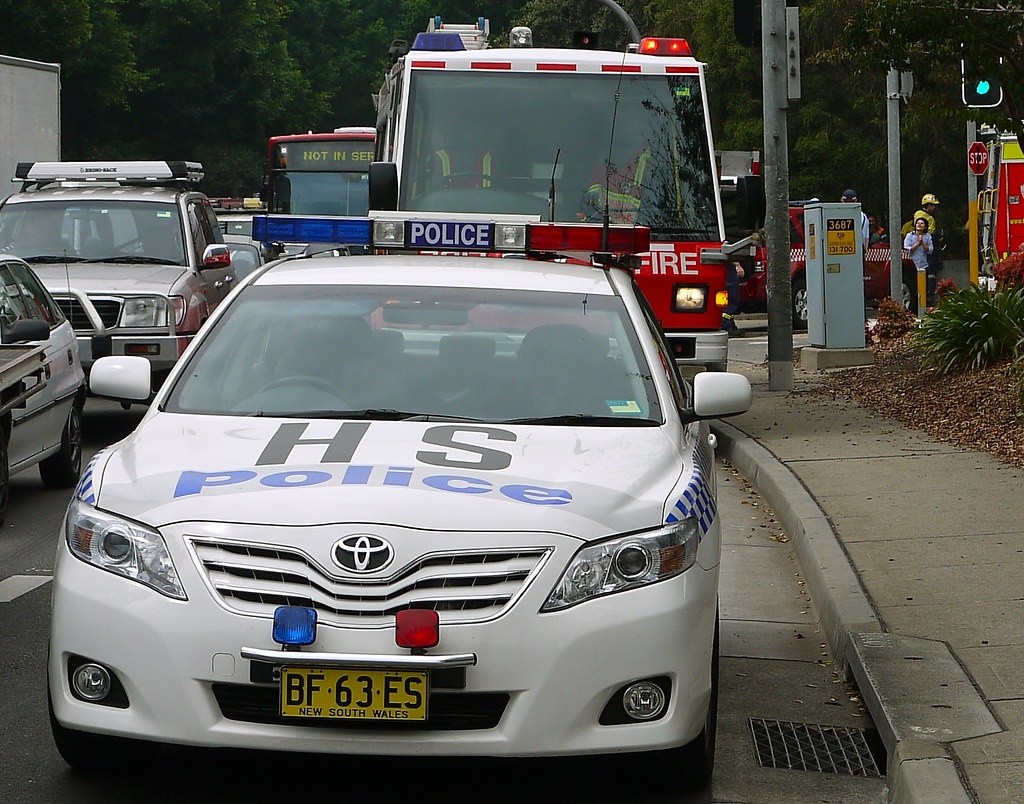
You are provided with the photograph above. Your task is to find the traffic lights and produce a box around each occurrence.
[961,43,1004,109]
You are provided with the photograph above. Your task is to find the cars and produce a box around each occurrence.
[0,253,88,503]
[221,233,269,281]
[49,216,752,802]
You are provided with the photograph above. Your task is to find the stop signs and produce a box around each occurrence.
[969,142,989,176]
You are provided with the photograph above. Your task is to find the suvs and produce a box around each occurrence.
[0,160,240,390]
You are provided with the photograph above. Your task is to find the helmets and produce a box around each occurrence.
[922,194,939,204]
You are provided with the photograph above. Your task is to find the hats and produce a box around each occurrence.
[842,190,856,203]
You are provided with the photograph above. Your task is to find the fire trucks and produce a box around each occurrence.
[258,126,379,260]
[979,118,1024,288]
[368,17,766,383]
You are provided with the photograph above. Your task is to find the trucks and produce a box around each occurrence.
[0,56,61,200]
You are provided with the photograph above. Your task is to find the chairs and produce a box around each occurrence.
[250,325,619,416]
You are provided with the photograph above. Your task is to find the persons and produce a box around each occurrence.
[841,190,869,320]
[584,125,656,224]
[721,261,745,333]
[914,194,942,306]
[904,218,933,315]
[867,214,886,239]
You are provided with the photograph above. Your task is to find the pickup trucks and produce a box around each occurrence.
[714,198,916,333]
